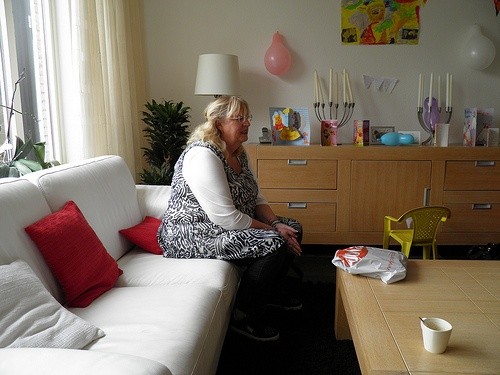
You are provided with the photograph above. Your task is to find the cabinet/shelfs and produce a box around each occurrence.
[245,143,500,246]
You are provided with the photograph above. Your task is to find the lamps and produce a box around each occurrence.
[194,54,241,98]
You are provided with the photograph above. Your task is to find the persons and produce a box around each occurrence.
[157,95,305,341]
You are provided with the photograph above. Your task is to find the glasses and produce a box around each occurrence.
[230,113,252,123]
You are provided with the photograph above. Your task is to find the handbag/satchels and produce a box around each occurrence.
[331,246,408,284]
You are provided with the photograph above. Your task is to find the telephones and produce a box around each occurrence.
[258,127,271,144]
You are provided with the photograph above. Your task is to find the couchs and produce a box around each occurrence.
[0,155,247,375]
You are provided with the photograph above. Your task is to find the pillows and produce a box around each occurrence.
[0,260,105,350]
[118,216,163,255]
[25,201,123,308]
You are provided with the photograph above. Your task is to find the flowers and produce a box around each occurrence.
[1,68,61,179]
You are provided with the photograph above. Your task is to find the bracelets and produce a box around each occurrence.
[270,219,282,227]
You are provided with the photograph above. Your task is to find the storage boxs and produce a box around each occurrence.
[353,119,370,146]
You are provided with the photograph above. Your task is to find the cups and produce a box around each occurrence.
[420,317,453,353]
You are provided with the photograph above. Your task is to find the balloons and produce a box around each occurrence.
[264,32,291,76]
[380,132,414,145]
[463,24,496,70]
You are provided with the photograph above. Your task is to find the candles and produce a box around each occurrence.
[328,68,333,102]
[445,72,453,108]
[437,75,441,107]
[341,69,354,104]
[313,71,324,104]
[418,73,424,107]
[428,72,433,106]
[335,72,338,104]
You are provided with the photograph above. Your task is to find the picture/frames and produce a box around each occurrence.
[371,126,394,145]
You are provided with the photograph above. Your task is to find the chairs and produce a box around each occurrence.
[383,206,451,260]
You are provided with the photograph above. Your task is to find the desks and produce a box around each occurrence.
[335,259,500,375]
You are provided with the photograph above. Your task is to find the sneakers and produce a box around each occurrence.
[230,318,280,341]
[266,292,303,310]
